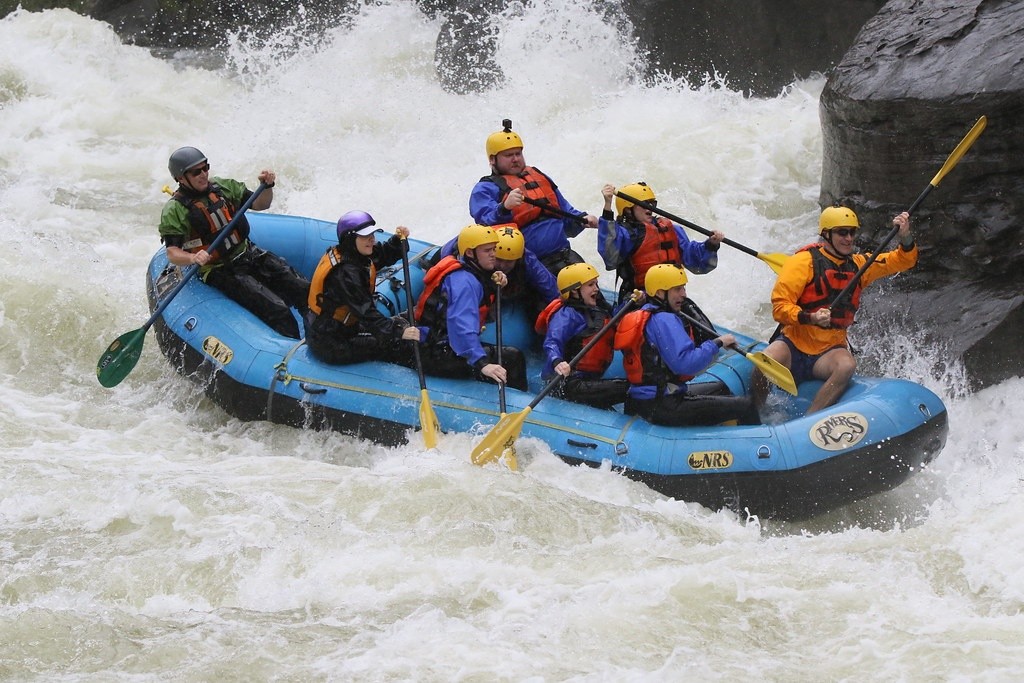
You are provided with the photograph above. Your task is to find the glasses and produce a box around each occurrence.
[190,164,212,176]
[826,229,857,238]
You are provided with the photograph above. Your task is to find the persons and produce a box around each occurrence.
[413,224,529,393]
[614,264,762,426]
[535,262,644,409]
[303,210,420,371]
[426,223,560,352]
[763,206,917,414]
[597,181,723,342]
[158,146,310,339]
[469,131,612,312]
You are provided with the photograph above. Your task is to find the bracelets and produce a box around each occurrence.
[265,182,274,189]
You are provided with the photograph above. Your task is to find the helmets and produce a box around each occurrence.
[557,263,600,301]
[615,182,657,216]
[495,226,524,261]
[457,224,499,256]
[336,210,376,239]
[485,131,524,161]
[818,206,861,235]
[168,146,209,183]
[644,263,688,297]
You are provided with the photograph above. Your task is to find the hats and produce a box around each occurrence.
[354,225,384,236]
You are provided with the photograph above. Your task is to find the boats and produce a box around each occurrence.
[143,210,951,522]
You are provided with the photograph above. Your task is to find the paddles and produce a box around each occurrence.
[610,184,794,276]
[397,226,442,447]
[469,271,647,473]
[640,293,802,396]
[515,193,603,229]
[97,177,271,389]
[825,115,991,316]
